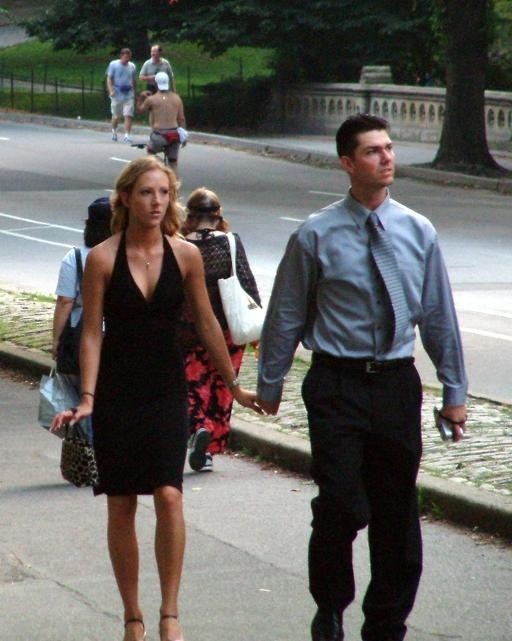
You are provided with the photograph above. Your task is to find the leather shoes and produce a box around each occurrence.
[311,606,344,641]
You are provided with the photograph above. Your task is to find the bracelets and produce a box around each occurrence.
[226,378,240,390]
[79,391,97,401]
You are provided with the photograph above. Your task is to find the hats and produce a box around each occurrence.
[156,70,171,92]
[88,195,112,221]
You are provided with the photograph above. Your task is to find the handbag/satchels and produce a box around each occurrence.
[163,130,181,145]
[39,373,88,437]
[57,326,81,374]
[60,440,100,487]
[118,84,133,94]
[217,274,266,346]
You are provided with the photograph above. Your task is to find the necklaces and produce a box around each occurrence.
[159,96,169,101]
[128,239,161,269]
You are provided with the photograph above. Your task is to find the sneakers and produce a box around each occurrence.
[189,427,215,472]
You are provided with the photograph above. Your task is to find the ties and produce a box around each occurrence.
[367,212,409,354]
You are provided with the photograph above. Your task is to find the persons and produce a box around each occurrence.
[47,196,117,455]
[136,72,187,196]
[254,114,468,640]
[103,47,138,147]
[49,158,258,640]
[174,186,265,476]
[139,45,177,102]
